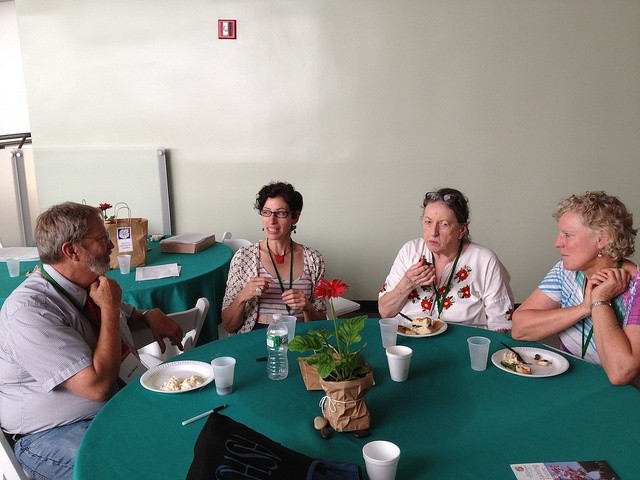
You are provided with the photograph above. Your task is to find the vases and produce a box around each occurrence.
[317,369,376,432]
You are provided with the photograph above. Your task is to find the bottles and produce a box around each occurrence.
[265,313,289,382]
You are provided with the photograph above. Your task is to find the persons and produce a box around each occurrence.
[507,193,640,385]
[378,188,514,336]
[221,181,327,334]
[0,202,183,480]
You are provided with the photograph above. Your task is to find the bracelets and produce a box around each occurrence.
[589,300,612,309]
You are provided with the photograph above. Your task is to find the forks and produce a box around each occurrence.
[500,339,532,367]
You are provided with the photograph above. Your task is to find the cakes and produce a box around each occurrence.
[501,351,520,369]
[398,316,444,338]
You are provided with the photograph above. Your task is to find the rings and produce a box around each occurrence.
[255,286,262,291]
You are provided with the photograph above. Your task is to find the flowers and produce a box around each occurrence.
[287,276,368,380]
[98,203,115,220]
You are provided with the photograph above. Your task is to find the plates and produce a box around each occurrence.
[491,346,571,379]
[139,360,214,394]
[393,314,449,338]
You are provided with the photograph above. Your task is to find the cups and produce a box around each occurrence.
[385,345,413,384]
[362,439,401,479]
[117,254,132,274]
[466,337,492,373]
[281,316,297,343]
[211,357,237,395]
[379,317,399,348]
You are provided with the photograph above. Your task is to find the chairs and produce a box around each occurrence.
[130,297,210,370]
[221,231,254,253]
[0,427,33,480]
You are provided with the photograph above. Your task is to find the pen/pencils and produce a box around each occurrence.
[181,404,231,425]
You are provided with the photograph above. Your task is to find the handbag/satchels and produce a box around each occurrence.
[186,412,363,480]
[297,341,375,391]
[105,202,148,270]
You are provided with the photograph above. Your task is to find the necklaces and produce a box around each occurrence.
[265,243,292,264]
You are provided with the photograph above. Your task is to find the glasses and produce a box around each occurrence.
[260,209,294,218]
[426,192,459,203]
[83,232,109,243]
